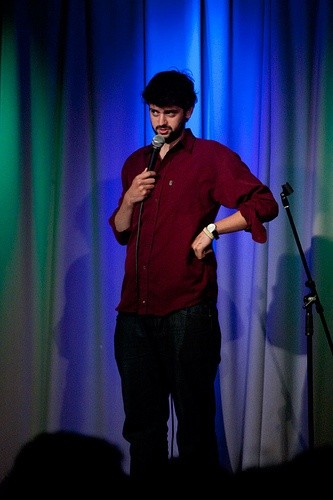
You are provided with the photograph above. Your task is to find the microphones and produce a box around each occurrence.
[145,135,165,179]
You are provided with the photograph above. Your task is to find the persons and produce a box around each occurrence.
[1,428,333,499]
[108,68,281,477]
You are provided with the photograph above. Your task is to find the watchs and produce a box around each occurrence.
[204,222,221,241]
